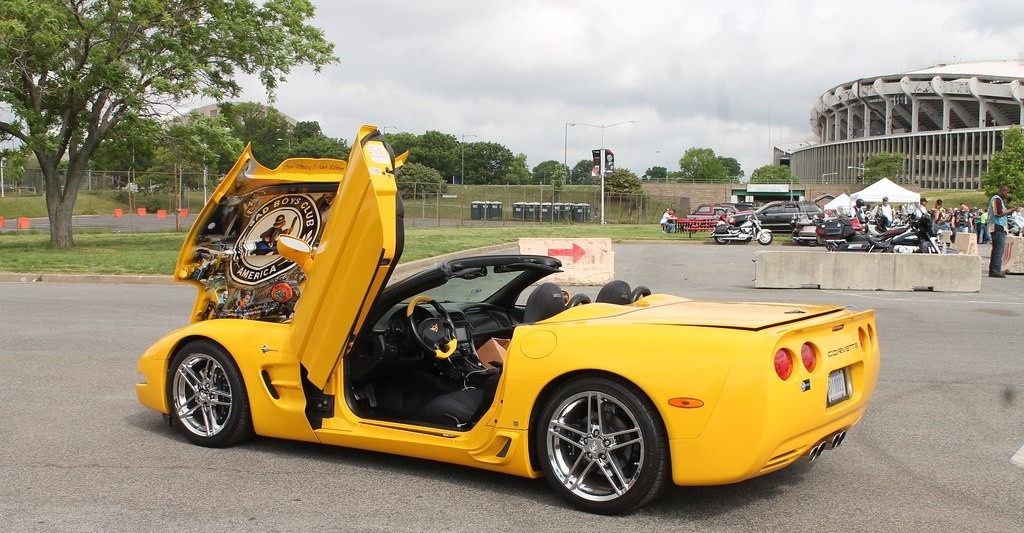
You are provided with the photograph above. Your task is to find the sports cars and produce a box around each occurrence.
[134,122,880,518]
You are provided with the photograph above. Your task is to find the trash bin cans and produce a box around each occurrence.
[514,201,592,221]
[471,201,502,220]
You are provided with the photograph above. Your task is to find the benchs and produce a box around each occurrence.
[685,224,716,238]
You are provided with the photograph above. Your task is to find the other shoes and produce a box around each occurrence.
[989,273,1006,278]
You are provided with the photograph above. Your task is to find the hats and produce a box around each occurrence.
[921,198,928,202]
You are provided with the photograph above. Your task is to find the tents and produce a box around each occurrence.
[824,177,920,219]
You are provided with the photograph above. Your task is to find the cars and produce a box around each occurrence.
[726,200,825,234]
[684,204,739,222]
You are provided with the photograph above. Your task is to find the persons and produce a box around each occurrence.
[719,209,731,222]
[661,208,677,233]
[987,186,1018,277]
[605,154,614,170]
[850,196,1024,258]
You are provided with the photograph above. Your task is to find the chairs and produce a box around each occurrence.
[424,284,564,425]
[596,281,632,305]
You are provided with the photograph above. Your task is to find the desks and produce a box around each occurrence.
[675,218,718,234]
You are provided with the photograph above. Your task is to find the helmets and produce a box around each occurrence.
[856,199,864,206]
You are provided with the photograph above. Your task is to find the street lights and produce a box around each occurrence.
[460,134,478,186]
[564,121,571,187]
[847,166,866,185]
[570,121,639,223]
[992,120,995,161]
[822,172,837,185]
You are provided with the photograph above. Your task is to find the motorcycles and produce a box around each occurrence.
[788,204,960,255]
[710,213,774,246]
